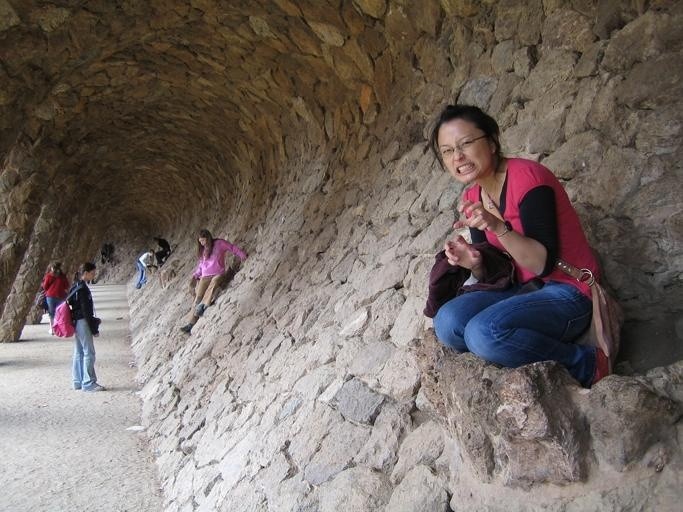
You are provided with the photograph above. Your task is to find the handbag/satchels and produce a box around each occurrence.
[35,290,47,310]
[578,265,624,358]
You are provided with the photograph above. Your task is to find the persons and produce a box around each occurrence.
[152,237,170,265]
[134,249,157,289]
[180,229,247,335]
[40,262,69,336]
[66,260,106,391]
[428,103,614,388]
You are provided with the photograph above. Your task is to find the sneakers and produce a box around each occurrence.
[195,305,204,317]
[94,385,106,391]
[181,325,191,332]
[593,349,613,388]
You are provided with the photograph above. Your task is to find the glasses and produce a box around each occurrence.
[437,133,485,161]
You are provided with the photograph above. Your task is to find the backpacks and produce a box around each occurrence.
[52,285,80,337]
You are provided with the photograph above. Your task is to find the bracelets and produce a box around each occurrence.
[494,220,512,237]
